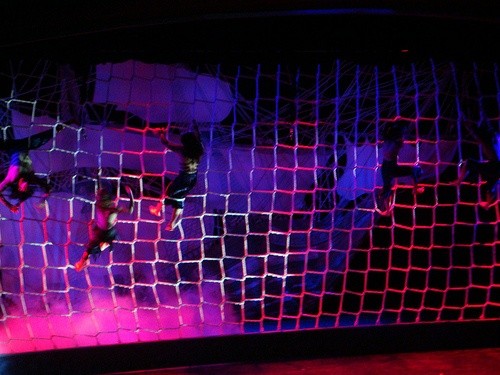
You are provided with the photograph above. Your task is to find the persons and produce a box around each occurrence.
[73,168,135,272]
[458,94,500,198]
[148,117,205,231]
[0,122,64,212]
[377,110,423,200]
